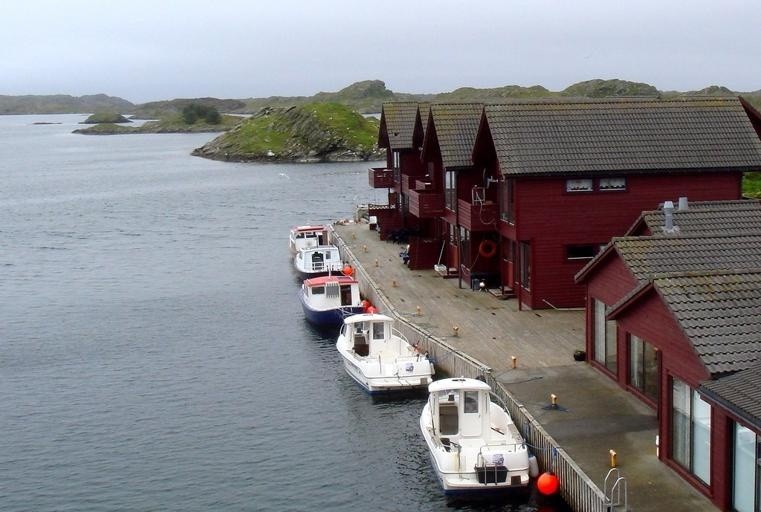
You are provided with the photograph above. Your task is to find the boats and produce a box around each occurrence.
[418,375,540,501]
[289,224,346,276]
[298,277,370,331]
[334,312,436,392]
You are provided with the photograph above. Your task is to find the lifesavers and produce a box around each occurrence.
[479,239,497,257]
